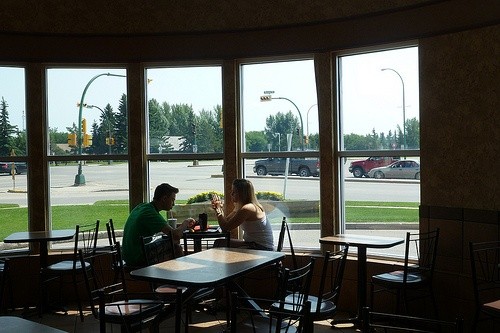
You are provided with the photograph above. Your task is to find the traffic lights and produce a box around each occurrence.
[147,77,153,84]
[218,107,223,128]
[76,101,87,108]
[260,95,272,102]
[81,118,87,134]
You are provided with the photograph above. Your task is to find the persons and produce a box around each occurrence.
[122,183,196,266]
[212,178,273,251]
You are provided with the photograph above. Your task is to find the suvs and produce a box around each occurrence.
[348,156,401,178]
[253,149,321,178]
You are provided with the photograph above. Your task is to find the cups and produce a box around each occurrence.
[199,213,207,231]
[168,218,177,229]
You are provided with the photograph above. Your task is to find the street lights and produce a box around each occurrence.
[381,69,405,138]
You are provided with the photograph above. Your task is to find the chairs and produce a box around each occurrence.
[0,216,500,333]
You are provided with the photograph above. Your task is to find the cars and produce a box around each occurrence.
[0,161,28,176]
[367,159,420,180]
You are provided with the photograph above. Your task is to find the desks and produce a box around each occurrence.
[162,225,232,316]
[3,229,76,317]
[129,247,287,333]
[319,233,404,333]
[0,315,70,333]
